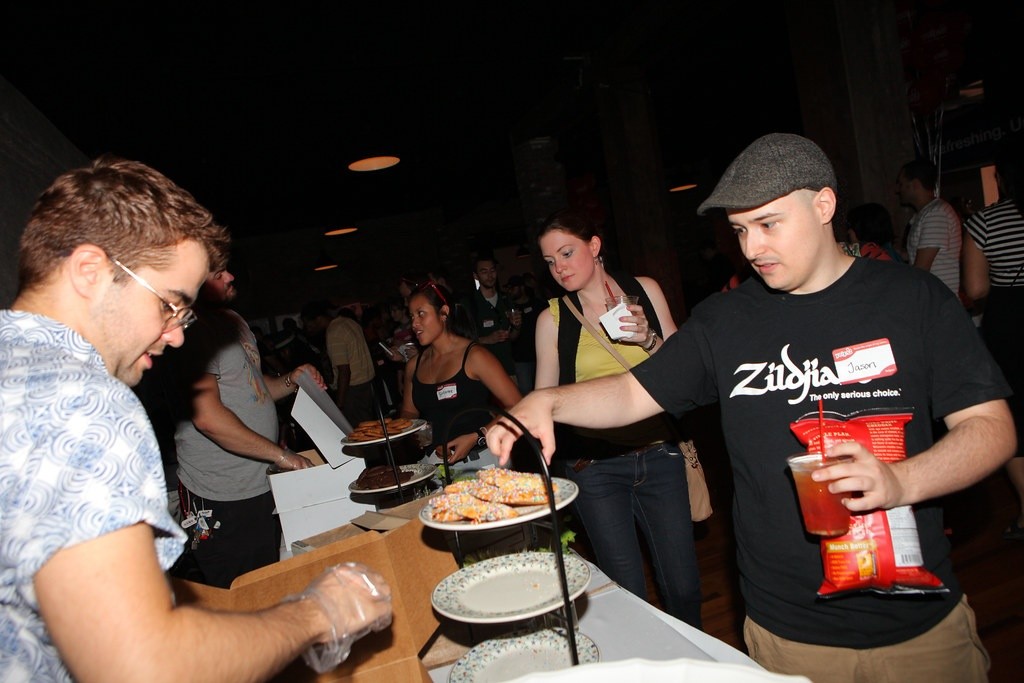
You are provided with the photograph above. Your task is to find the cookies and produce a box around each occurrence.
[348,417,414,441]
[357,464,414,489]
[430,467,559,522]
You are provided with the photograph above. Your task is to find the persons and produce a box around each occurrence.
[845,202,901,248]
[0,154,392,683]
[469,252,549,395]
[898,157,963,297]
[484,133,1018,683]
[965,140,1024,540]
[155,264,327,587]
[379,269,431,397]
[395,279,523,466]
[250,300,377,427]
[484,207,704,633]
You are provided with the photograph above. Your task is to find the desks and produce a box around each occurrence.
[425,533,721,683]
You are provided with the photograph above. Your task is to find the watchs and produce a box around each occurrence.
[476,430,486,446]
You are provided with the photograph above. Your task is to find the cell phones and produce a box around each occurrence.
[379,342,394,356]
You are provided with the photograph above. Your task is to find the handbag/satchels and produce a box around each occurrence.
[679,437,714,522]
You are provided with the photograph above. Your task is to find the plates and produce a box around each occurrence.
[348,464,437,494]
[430,554,590,623]
[418,477,579,531]
[446,625,598,682]
[341,418,427,445]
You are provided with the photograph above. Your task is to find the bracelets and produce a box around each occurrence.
[279,440,288,450]
[643,329,657,351]
[479,427,487,432]
[285,372,298,394]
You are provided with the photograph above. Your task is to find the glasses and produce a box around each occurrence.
[416,279,446,306]
[106,253,198,335]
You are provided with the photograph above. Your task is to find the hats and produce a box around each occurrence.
[698,133,839,217]
[502,275,522,288]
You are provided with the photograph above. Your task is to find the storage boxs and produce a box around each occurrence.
[173,369,459,683]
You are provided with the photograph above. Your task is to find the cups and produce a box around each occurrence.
[605,295,640,317]
[414,422,433,446]
[509,308,519,324]
[405,342,415,350]
[786,451,856,535]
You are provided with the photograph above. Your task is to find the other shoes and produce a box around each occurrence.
[1004,518,1024,538]
